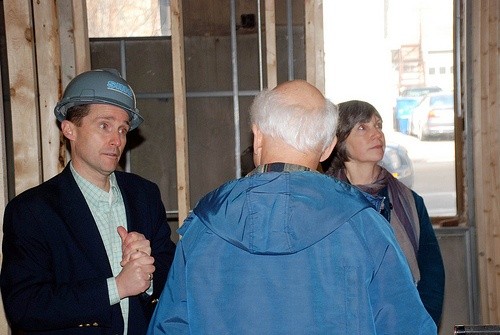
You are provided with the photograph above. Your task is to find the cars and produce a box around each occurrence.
[408,91,455,141]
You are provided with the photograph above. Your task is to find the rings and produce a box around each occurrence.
[148,272,152,282]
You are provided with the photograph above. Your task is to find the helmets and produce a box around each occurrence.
[54,69,145,132]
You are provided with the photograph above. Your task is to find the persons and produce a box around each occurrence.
[1,68,176,335]
[316,100,448,334]
[147,80,438,334]
